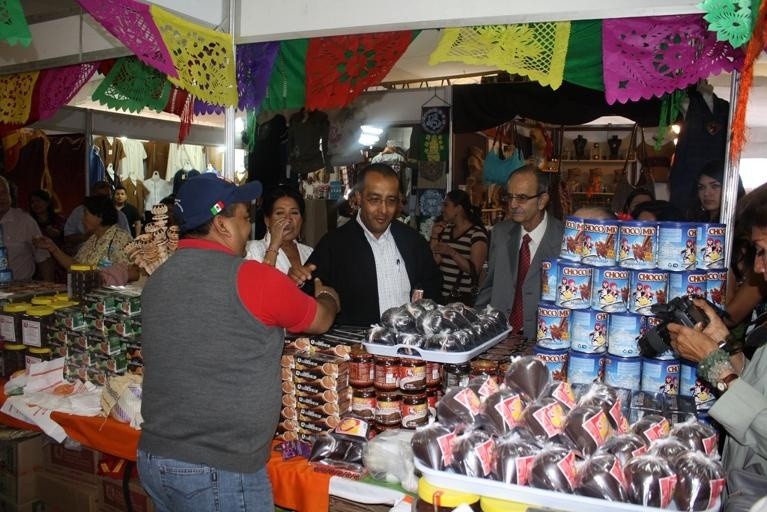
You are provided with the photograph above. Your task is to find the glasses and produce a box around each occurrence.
[500,190,547,203]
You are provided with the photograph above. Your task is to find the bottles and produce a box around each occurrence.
[608,134,622,160]
[572,135,587,159]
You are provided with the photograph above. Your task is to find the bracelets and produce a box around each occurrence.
[264,248,280,257]
[316,288,338,312]
[717,334,748,374]
[430,236,440,241]
[694,350,732,384]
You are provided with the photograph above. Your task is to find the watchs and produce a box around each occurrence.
[712,373,740,393]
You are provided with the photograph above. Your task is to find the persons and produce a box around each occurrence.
[133,165,345,512]
[668,180,765,510]
[571,204,618,221]
[471,163,565,346]
[244,183,314,277]
[428,187,488,307]
[283,160,444,321]
[622,188,656,214]
[630,198,679,222]
[693,159,765,373]
[0,173,178,286]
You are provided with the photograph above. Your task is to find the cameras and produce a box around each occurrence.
[638,291,710,361]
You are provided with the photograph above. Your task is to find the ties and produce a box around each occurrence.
[508,236,533,336]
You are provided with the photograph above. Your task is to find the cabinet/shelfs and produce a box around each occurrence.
[555,124,638,196]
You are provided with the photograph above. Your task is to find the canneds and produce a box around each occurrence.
[538,301,678,360]
[417,475,544,512]
[0,293,79,379]
[559,217,726,270]
[67,264,96,301]
[537,348,712,403]
[445,335,535,387]
[348,352,445,434]
[541,257,726,316]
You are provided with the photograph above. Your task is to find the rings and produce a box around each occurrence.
[278,222,282,228]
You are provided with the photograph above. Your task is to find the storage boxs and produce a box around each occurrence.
[0,493,42,512]
[0,434,42,502]
[44,440,100,490]
[97,476,153,512]
[37,472,99,512]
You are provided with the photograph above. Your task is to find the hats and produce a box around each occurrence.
[173,172,263,233]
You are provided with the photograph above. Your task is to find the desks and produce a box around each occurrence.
[0,359,420,512]
